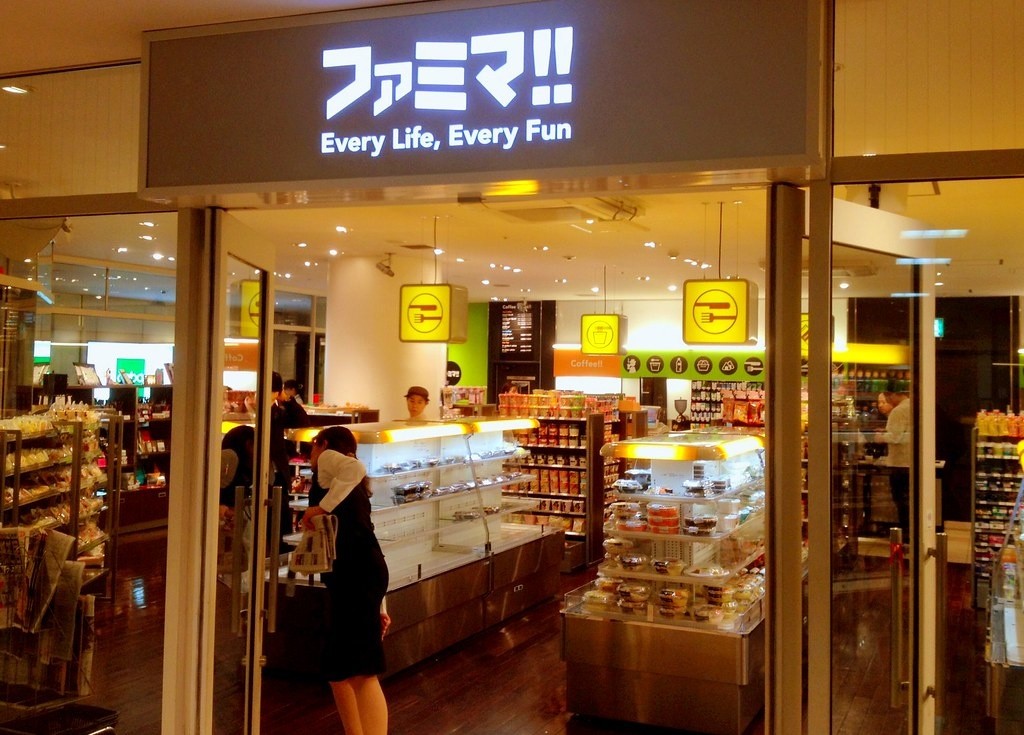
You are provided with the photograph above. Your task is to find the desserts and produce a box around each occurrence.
[583,469,764,620]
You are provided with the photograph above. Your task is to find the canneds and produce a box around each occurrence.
[975,471,1024,521]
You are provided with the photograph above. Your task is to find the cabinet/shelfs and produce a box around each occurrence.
[830,361,911,533]
[690,380,763,430]
[265,415,564,679]
[560,432,766,735]
[503,410,649,572]
[801,391,862,571]
[16,384,173,537]
[0,415,124,605]
[986,503,1024,718]
[971,427,1024,609]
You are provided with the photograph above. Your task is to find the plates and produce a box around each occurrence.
[683,565,730,578]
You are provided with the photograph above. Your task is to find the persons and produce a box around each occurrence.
[302,427,391,735]
[403,386,430,421]
[868,377,911,575]
[266,371,310,557]
[218,425,254,508]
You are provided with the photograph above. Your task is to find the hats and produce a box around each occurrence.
[404,387,430,402]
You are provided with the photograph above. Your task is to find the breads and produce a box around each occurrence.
[383,448,517,519]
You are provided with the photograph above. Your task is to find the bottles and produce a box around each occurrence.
[842,367,912,395]
[870,402,880,421]
[862,407,870,419]
[978,410,1024,438]
